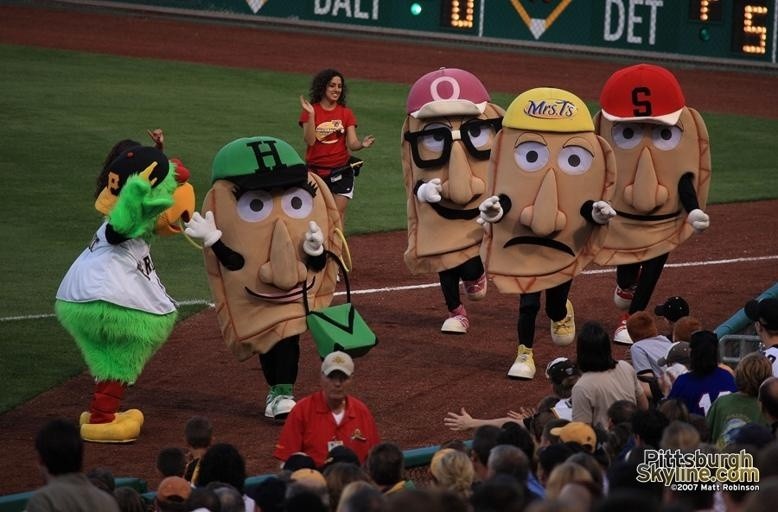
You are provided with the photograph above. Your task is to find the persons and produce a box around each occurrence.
[299,69,376,281]
[26,296,778,512]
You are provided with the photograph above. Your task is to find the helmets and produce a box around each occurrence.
[535,442,574,477]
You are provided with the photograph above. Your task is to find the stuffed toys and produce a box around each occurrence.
[52,129,201,444]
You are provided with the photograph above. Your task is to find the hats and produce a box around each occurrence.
[545,356,579,385]
[548,419,596,450]
[320,350,355,379]
[154,474,192,509]
[656,341,691,366]
[744,298,778,332]
[654,295,690,322]
